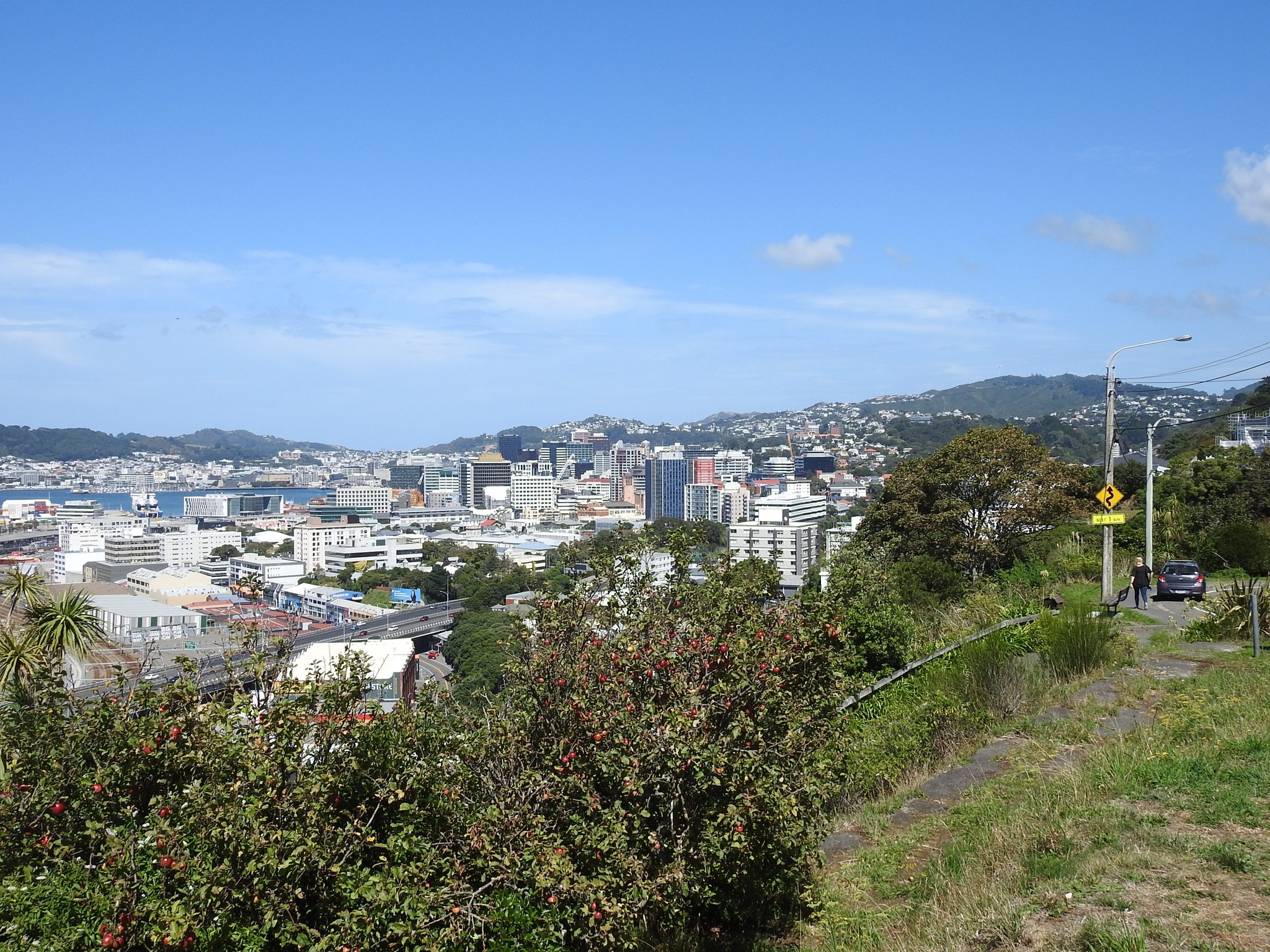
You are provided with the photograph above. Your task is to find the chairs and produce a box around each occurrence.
[1187,567,1195,573]
[1168,568,1176,574]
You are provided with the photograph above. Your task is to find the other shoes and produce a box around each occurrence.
[1144,605,1148,610]
[1134,606,1139,609]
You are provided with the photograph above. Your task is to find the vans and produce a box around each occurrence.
[388,625,398,631]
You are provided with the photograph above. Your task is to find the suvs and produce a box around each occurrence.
[1157,560,1210,602]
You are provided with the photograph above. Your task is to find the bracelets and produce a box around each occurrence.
[1131,583,1133,584]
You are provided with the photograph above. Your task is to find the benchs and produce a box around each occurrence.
[1098,585,1130,615]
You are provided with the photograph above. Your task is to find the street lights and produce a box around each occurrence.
[334,611,345,642]
[440,591,448,620]
[379,603,388,635]
[1146,417,1194,579]
[400,583,407,610]
[1101,335,1192,600]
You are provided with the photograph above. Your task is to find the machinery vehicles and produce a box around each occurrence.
[410,472,424,507]
[553,453,576,482]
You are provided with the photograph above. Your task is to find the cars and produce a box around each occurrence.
[323,620,334,624]
[357,621,366,626]
[425,651,439,659]
[359,630,368,636]
[442,606,450,610]
[449,613,455,617]
[350,625,359,630]
[420,616,429,621]
[430,605,439,609]
[169,676,179,683]
[145,674,159,680]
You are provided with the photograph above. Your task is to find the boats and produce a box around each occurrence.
[130,493,163,518]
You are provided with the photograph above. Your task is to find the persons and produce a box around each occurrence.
[1130,557,1154,610]
[410,590,416,603]
